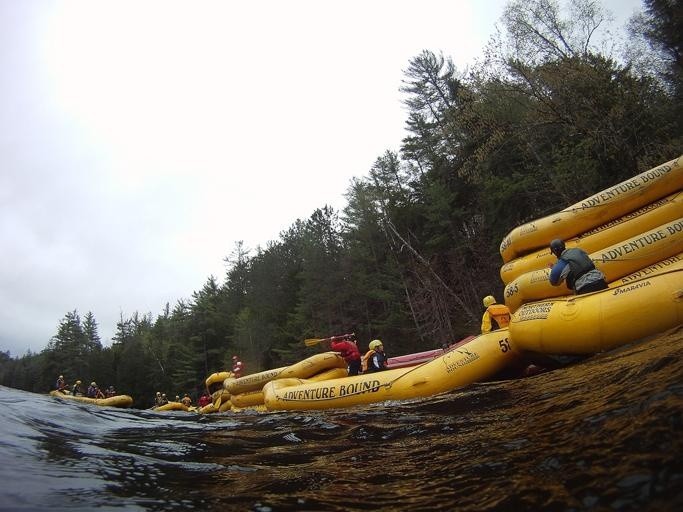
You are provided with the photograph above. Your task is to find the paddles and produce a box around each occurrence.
[214,369,233,408]
[304,332,355,347]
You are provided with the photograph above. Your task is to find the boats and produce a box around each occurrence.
[498,155,682,263]
[230,368,348,407]
[149,369,235,413]
[50,391,132,408]
[507,253,682,355]
[260,327,518,411]
[222,351,348,394]
[229,405,266,414]
[499,192,682,286]
[503,218,682,313]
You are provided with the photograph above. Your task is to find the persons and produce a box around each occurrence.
[56,375,117,400]
[549,239,607,294]
[230,355,245,379]
[150,391,213,408]
[480,295,510,334]
[329,334,360,376]
[362,339,387,373]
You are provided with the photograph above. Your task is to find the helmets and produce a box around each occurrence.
[550,238,565,254]
[369,339,383,350]
[76,380,81,385]
[59,375,63,379]
[344,333,354,341]
[90,381,97,387]
[483,295,497,308]
